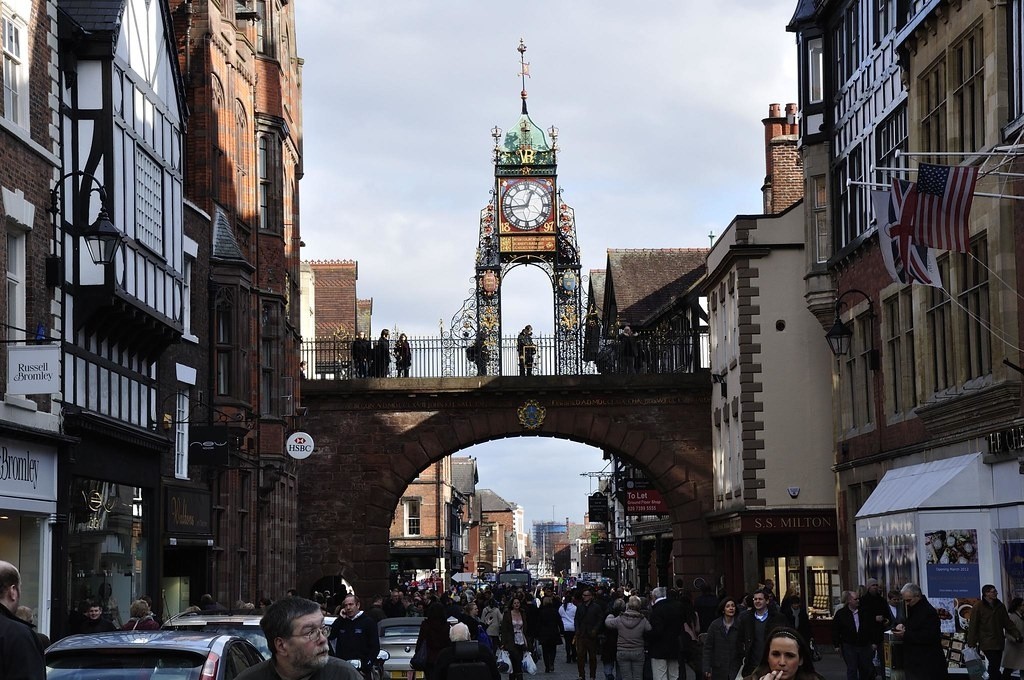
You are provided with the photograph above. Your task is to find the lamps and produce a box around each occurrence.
[45,170,127,289]
[823,289,878,367]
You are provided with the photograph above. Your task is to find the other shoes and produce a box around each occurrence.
[545,667,549,673]
[590,678,596,680]
[576,677,585,680]
[550,665,554,671]
[607,674,614,680]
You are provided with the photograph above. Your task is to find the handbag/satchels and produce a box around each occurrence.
[477,626,493,650]
[410,641,429,671]
[961,647,987,680]
[521,651,538,676]
[538,644,543,660]
[496,649,513,674]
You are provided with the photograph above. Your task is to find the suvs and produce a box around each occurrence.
[43,629,266,680]
[162,605,272,662]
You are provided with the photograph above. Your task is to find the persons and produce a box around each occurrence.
[299,324,638,380]
[0,552,1024,680]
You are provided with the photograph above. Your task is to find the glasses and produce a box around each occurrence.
[753,598,763,601]
[583,594,590,596]
[291,625,332,642]
[872,584,878,586]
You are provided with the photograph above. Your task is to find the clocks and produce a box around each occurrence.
[503,181,553,230]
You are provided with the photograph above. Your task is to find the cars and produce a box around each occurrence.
[376,617,428,679]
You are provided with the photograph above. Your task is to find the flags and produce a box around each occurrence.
[582,276,600,362]
[601,255,618,339]
[872,162,978,290]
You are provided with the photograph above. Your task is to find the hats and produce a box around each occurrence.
[867,578,878,587]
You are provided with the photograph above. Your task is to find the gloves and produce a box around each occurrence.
[1016,636,1023,643]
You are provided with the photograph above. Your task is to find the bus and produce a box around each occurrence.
[496,570,531,593]
